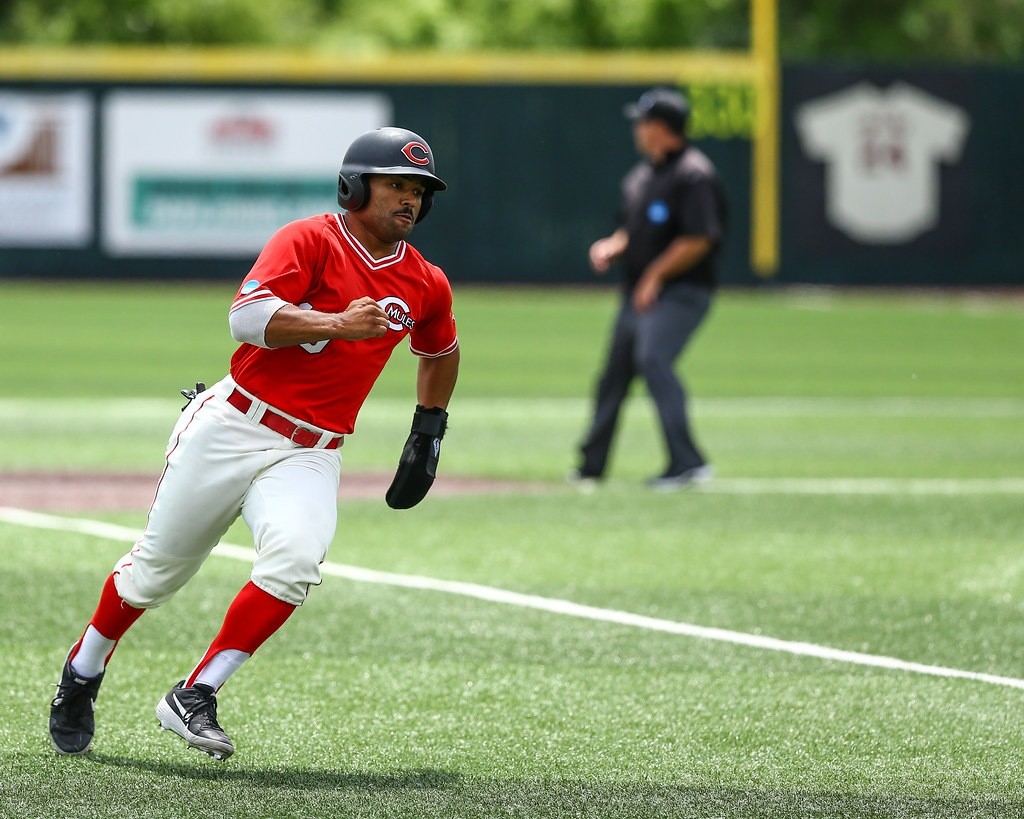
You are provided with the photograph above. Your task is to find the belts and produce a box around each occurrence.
[228,387,345,451]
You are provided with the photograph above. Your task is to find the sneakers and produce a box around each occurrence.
[156,679,233,764]
[47,639,108,755]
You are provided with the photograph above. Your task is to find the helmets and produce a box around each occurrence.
[337,127,448,226]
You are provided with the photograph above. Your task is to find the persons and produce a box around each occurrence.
[553,92,727,485]
[50,127,461,760]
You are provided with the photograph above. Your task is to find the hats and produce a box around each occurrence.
[626,91,690,137]
